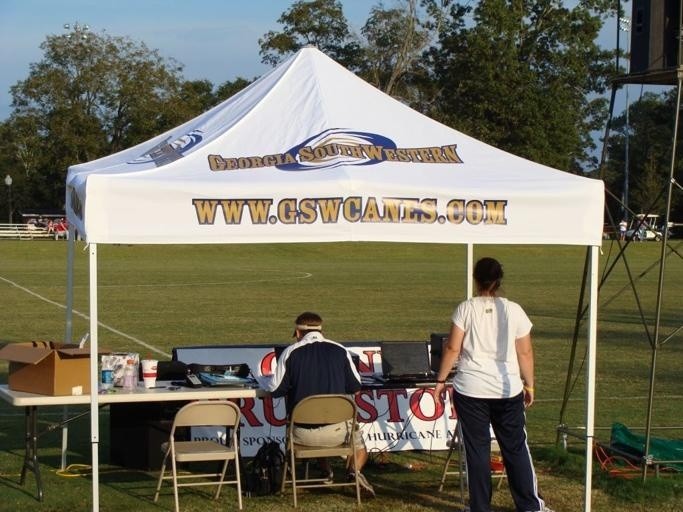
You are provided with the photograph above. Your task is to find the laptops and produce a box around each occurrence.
[379,340,439,382]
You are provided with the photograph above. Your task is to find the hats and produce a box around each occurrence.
[292,325,321,337]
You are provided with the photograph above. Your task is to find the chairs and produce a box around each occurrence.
[279,393,364,508]
[440,413,528,501]
[156,401,250,511]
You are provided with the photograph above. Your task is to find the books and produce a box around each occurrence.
[201,371,250,386]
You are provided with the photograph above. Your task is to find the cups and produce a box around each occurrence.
[140,359,159,389]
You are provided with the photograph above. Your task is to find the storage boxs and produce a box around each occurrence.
[0,339,110,395]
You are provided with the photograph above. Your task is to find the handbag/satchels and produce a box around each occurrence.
[243,441,285,497]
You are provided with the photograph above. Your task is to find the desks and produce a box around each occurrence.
[2,379,272,501]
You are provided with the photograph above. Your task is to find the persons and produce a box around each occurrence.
[619,216,647,242]
[47,218,69,241]
[434,257,560,512]
[268,312,378,500]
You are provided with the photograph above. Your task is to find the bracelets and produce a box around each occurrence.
[436,379,445,383]
[523,387,535,392]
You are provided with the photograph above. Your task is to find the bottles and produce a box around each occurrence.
[100,353,115,393]
[123,359,137,394]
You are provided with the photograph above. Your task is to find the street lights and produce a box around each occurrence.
[4,174,12,223]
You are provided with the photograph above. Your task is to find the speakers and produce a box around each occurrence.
[430,333,459,373]
[631,0,683,72]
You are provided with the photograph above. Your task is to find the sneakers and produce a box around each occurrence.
[320,468,376,502]
